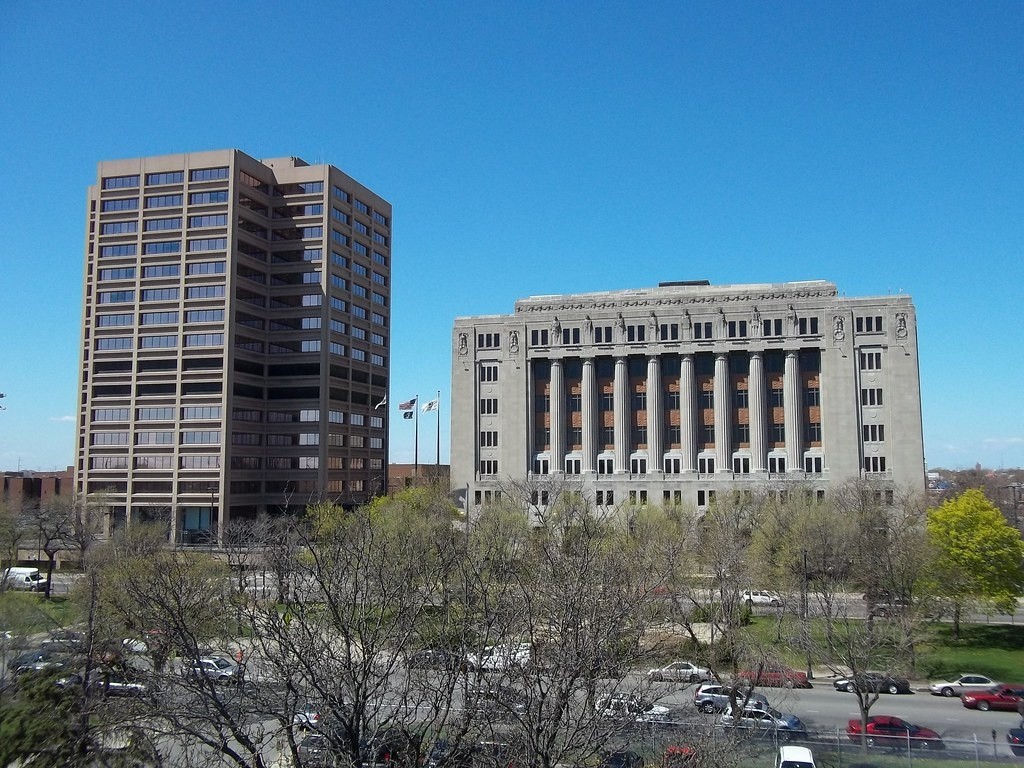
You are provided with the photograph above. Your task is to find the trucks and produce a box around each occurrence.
[5,567,49,593]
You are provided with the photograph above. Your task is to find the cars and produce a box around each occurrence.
[650,661,712,683]
[9,618,248,702]
[733,661,808,690]
[844,715,945,750]
[285,692,705,767]
[405,637,557,677]
[1006,722,1024,755]
[929,671,1000,697]
[833,670,910,694]
[960,682,1024,712]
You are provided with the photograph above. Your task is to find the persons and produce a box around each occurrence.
[746,304,763,337]
[580,313,594,342]
[713,306,728,339]
[549,315,563,345]
[783,303,800,336]
[612,312,625,344]
[679,308,692,341]
[644,309,659,342]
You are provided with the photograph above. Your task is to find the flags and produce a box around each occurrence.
[421,400,438,414]
[398,398,417,411]
[403,410,414,420]
[374,395,387,410]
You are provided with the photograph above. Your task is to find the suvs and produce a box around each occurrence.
[722,700,803,737]
[741,589,786,607]
[774,746,817,768]
[694,682,768,715]
